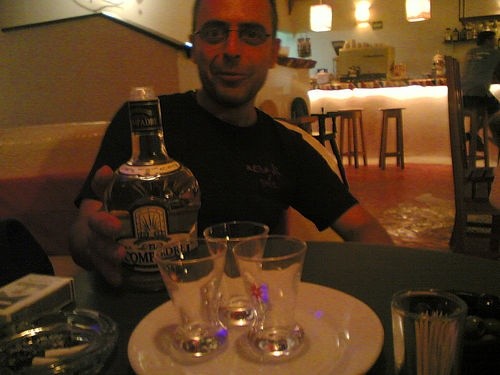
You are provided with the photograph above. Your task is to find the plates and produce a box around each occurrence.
[128,281,385,375]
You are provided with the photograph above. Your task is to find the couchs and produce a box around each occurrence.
[0,120,345,257]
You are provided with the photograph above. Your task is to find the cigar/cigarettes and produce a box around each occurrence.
[45,343,89,358]
[32,357,60,366]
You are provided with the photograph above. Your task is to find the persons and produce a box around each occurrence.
[459,30,500,149]
[67,0,396,287]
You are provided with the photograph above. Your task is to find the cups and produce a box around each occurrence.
[389,288,468,374]
[231,234,308,362]
[204,220,270,330]
[154,239,229,360]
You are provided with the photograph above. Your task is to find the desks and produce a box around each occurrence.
[0,240,500,375]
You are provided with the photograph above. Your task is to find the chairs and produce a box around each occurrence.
[445,55,500,252]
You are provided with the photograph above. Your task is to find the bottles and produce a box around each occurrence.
[432,53,446,77]
[100,87,201,292]
[444,26,478,41]
[297,38,311,57]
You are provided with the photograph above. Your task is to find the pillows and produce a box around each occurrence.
[0,219,56,287]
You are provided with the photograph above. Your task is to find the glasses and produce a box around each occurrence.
[193,20,276,45]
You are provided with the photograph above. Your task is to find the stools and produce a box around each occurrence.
[461,97,489,169]
[272,108,407,194]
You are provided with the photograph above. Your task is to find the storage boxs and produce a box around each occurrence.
[0,273,76,339]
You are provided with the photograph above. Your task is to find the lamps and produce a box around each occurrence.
[405,0,430,23]
[309,0,332,32]
